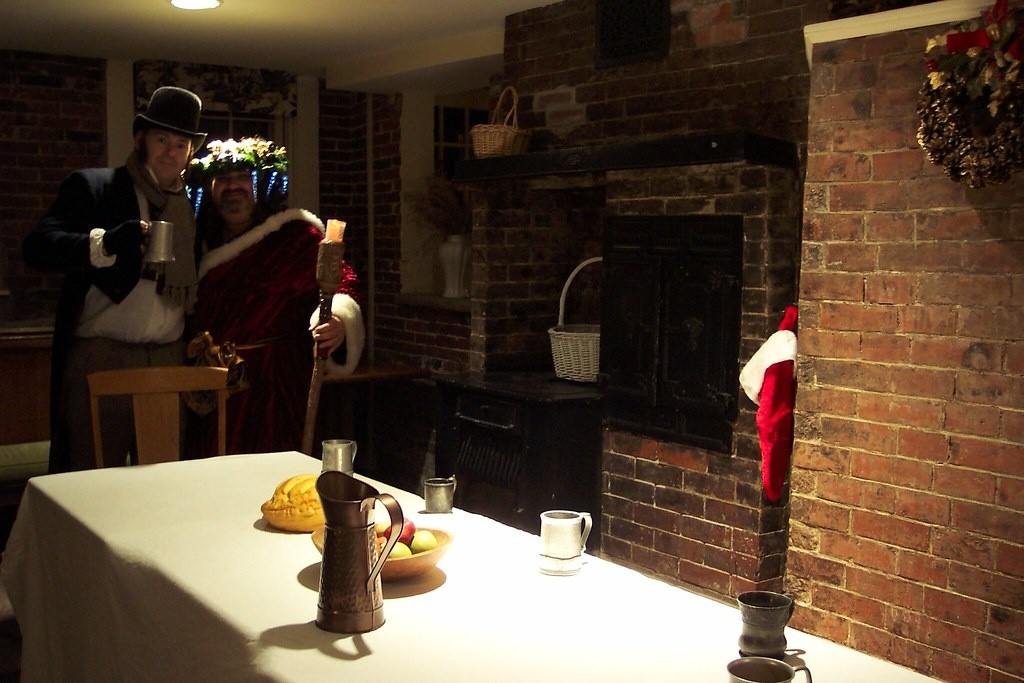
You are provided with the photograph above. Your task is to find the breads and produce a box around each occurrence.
[261,473,329,532]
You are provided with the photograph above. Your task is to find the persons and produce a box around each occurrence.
[18,85,211,473]
[185,135,366,461]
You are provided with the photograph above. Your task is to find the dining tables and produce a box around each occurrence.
[0,450,950,683]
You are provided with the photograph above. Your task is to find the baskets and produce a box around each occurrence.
[471,85,531,158]
[547,257,602,382]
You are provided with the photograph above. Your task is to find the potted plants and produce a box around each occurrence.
[403,174,474,299]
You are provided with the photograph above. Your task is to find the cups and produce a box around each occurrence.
[727,657,813,683]
[738,591,795,661]
[424,475,457,514]
[322,440,357,477]
[538,510,592,576]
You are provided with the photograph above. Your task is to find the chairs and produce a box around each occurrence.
[86,366,230,467]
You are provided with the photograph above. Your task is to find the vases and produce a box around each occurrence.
[916,78,1024,187]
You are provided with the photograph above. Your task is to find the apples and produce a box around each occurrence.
[372,520,439,560]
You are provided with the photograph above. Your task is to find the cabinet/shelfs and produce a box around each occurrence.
[0,317,55,446]
[601,216,743,455]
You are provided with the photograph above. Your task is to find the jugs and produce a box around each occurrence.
[315,471,404,635]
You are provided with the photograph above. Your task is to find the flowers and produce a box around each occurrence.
[912,0,1024,116]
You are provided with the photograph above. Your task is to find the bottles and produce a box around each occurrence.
[142,221,174,281]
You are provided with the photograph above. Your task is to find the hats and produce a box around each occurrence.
[132,87,207,154]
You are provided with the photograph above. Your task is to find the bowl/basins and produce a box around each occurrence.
[311,527,453,583]
